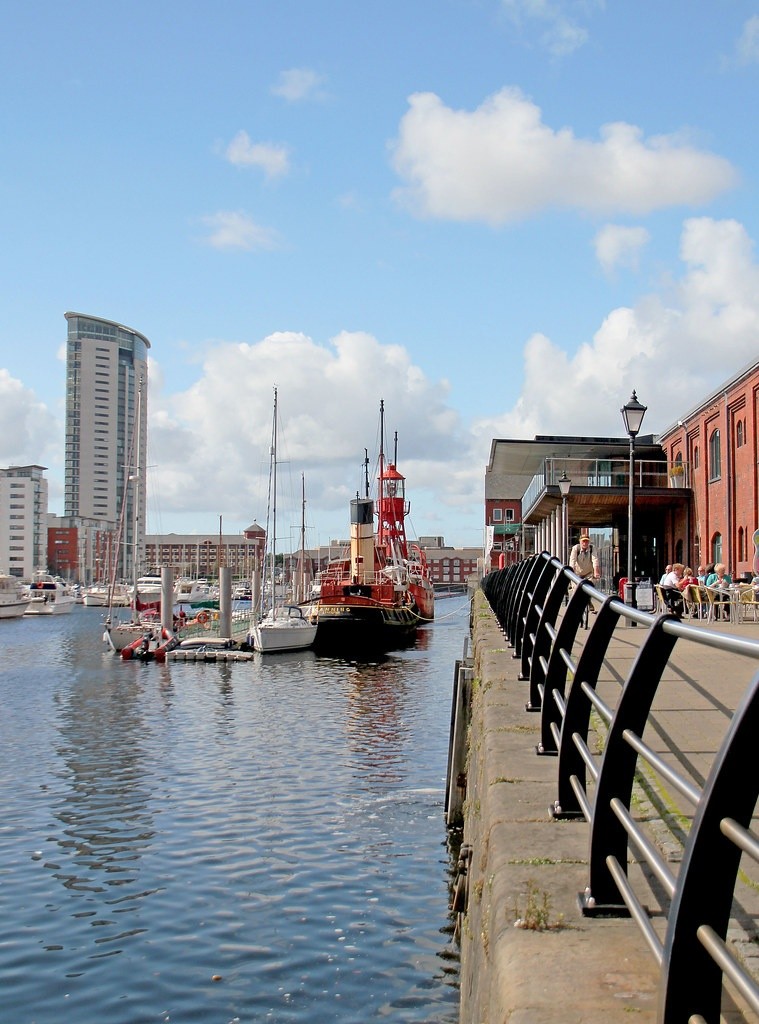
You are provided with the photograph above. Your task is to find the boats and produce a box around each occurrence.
[0,571,31,620]
[76,575,274,607]
[19,568,77,614]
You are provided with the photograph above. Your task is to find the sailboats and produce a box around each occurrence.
[252,387,317,652]
[309,397,433,641]
[285,471,317,618]
[102,373,185,653]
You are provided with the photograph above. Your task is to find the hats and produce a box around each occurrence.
[580,534,590,541]
[698,565,705,570]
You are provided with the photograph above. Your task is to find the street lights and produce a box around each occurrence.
[620,388,647,627]
[558,470,572,563]
[504,531,519,566]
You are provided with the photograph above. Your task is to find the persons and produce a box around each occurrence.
[658,562,733,621]
[737,569,759,622]
[569,534,599,614]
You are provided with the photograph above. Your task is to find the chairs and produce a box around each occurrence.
[704,585,735,625]
[740,585,759,624]
[654,584,668,615]
[688,584,715,622]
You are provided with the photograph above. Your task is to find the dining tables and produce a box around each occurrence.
[729,583,754,625]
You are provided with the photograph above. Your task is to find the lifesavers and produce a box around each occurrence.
[37,582,42,588]
[197,613,207,623]
[498,553,503,569]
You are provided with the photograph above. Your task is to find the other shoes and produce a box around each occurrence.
[589,604,594,610]
[676,614,684,618]
